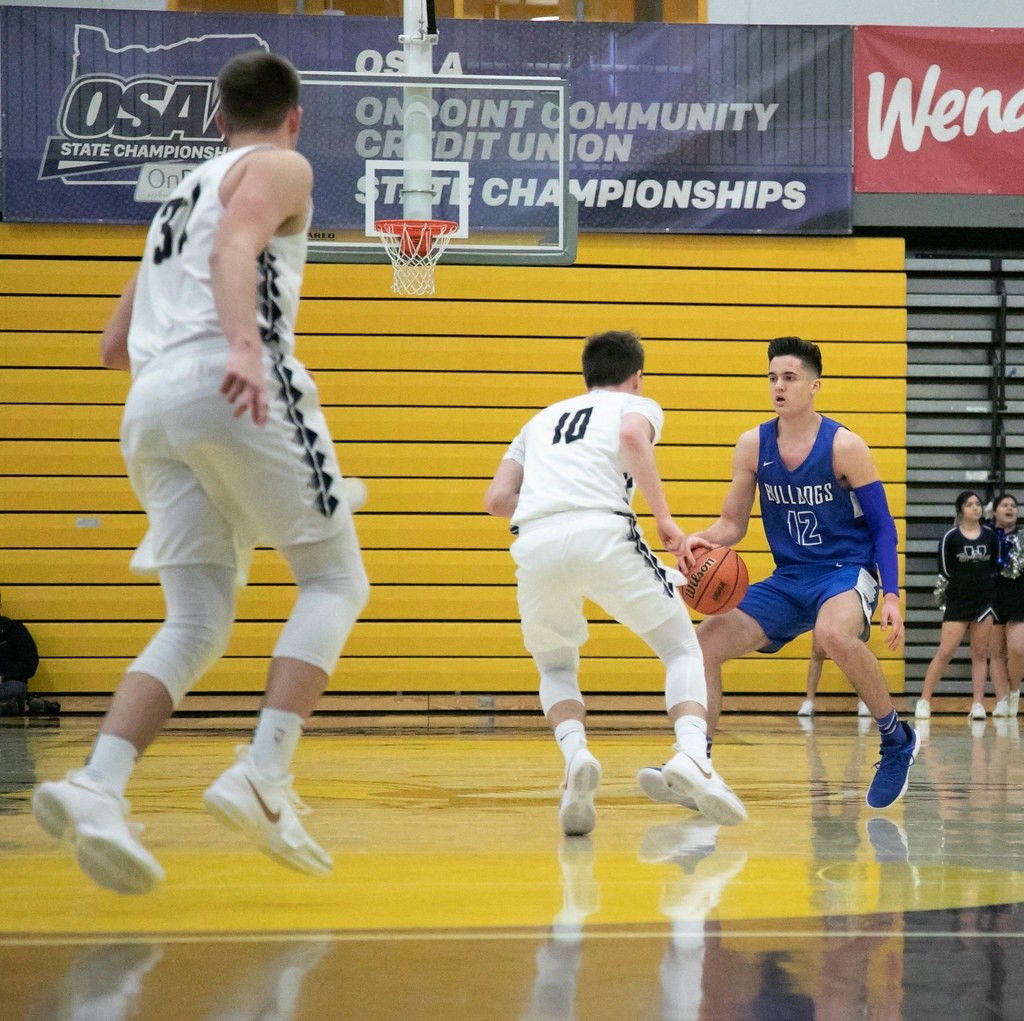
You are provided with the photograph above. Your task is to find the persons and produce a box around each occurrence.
[0,616,39,713]
[796,620,877,716]
[486,329,750,835]
[977,493,1023,718]
[29,50,370,901]
[639,334,921,818]
[915,491,1006,720]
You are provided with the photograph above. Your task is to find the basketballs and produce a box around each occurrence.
[676,543,749,616]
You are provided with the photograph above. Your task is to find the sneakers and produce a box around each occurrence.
[200,743,334,879]
[992,718,1009,737]
[865,817,918,891]
[866,719,921,810]
[32,771,163,897]
[857,718,871,733]
[558,749,602,837]
[915,720,931,747]
[58,943,165,1021]
[857,701,873,717]
[638,754,712,813]
[658,848,748,921]
[1007,689,1020,717]
[915,698,931,719]
[635,815,720,875]
[1008,718,1019,740]
[797,701,816,716]
[556,835,600,926]
[661,741,747,826]
[968,703,986,719]
[992,700,1008,717]
[797,717,815,736]
[967,720,987,738]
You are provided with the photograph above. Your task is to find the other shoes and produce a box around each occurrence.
[0,695,61,728]
